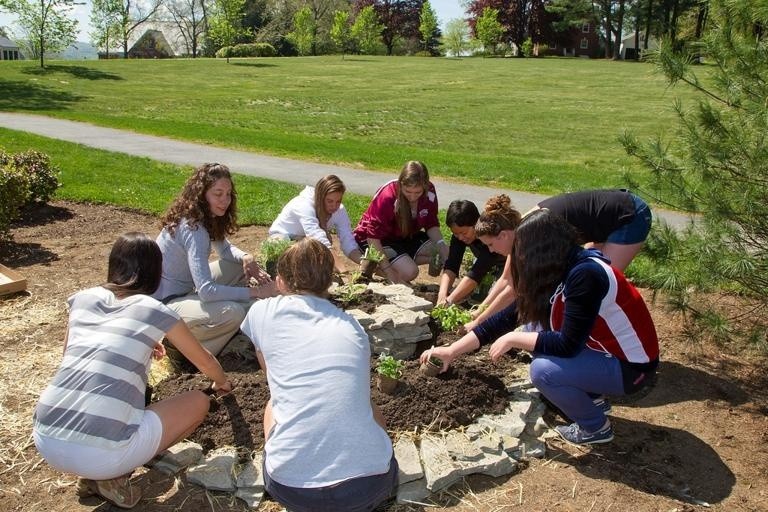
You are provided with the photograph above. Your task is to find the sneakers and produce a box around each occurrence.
[162,339,200,375]
[591,398,612,416]
[75,478,99,496]
[555,417,614,445]
[96,474,144,508]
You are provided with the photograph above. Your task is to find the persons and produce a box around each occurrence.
[239,237,400,512]
[354,160,451,290]
[418,208,660,448]
[267,174,380,276]
[434,199,508,318]
[456,188,651,338]
[148,161,282,371]
[32,233,239,508]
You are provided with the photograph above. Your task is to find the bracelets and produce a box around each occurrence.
[381,261,391,271]
[256,286,263,298]
[444,298,453,306]
[437,239,445,246]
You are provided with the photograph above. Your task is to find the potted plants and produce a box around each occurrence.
[376,351,405,394]
[419,357,445,377]
[359,244,385,274]
[428,243,443,277]
